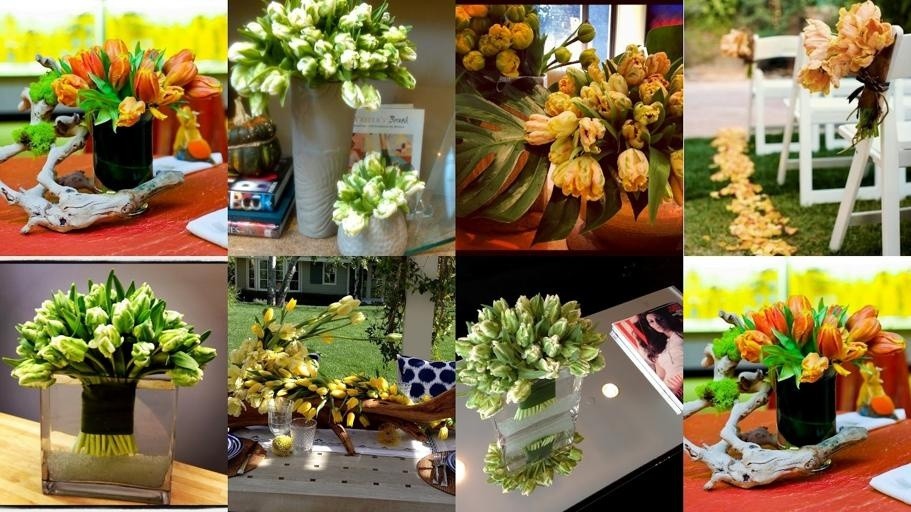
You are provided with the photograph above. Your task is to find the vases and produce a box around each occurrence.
[496,410,582,475]
[89,114,153,192]
[580,146,682,246]
[489,367,579,440]
[288,74,355,237]
[38,369,180,506]
[457,87,552,235]
[775,371,836,450]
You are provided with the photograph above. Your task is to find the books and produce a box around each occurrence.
[609,302,683,415]
[228,157,295,238]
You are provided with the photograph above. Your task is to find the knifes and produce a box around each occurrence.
[237,437,260,475]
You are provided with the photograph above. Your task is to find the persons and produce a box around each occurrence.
[349,132,412,170]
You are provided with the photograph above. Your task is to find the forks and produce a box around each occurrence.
[431,450,450,487]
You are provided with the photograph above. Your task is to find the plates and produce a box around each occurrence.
[446,449,456,473]
[227,432,244,461]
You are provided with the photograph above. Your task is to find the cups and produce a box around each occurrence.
[268,396,296,435]
[289,417,318,453]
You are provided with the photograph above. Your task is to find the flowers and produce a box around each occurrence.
[456,283,609,419]
[455,41,683,248]
[330,144,426,240]
[25,29,224,129]
[794,0,899,155]
[710,287,905,392]
[456,5,604,98]
[228,294,455,449]
[719,30,754,78]
[480,431,586,497]
[228,1,419,115]
[1,267,222,459]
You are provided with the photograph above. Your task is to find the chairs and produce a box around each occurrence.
[745,23,909,256]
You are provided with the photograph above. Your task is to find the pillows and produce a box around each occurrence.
[394,349,455,408]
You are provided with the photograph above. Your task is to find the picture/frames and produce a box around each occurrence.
[334,208,408,256]
